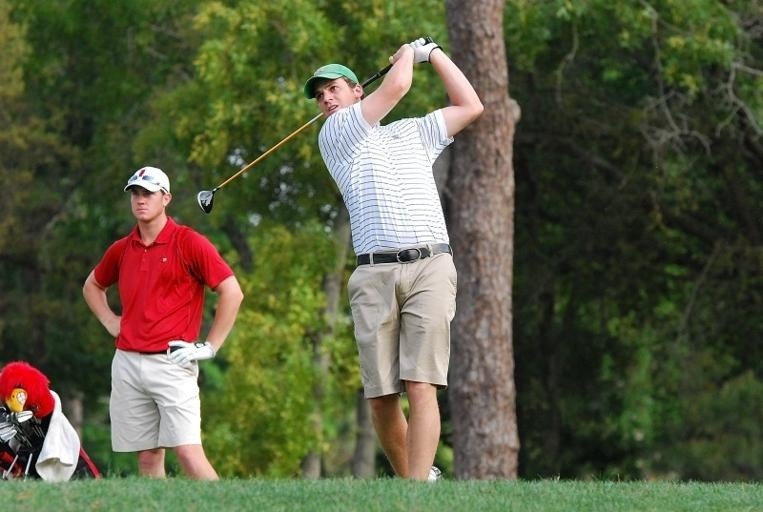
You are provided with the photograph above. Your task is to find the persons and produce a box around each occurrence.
[81,166,246,482]
[302,34,486,481]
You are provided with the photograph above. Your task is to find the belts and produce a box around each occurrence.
[141,344,184,354]
[354,241,455,266]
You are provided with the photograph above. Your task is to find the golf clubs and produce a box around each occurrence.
[0,388,28,455]
[196,36,433,213]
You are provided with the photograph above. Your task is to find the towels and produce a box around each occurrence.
[35,390,80,483]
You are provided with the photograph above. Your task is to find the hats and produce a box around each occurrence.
[303,62,362,99]
[122,164,171,200]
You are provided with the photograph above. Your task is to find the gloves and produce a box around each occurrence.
[166,339,217,367]
[406,33,443,65]
